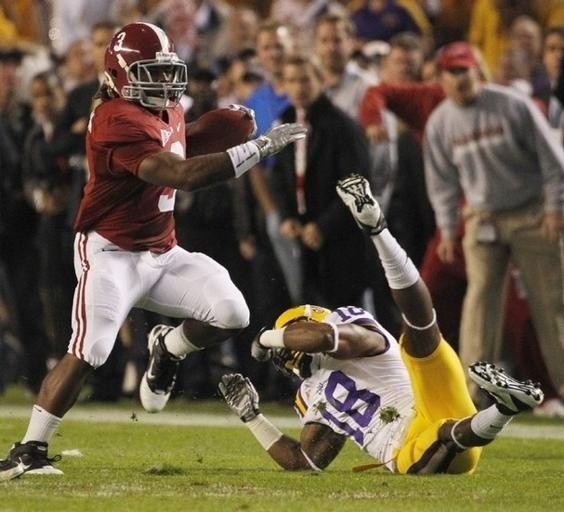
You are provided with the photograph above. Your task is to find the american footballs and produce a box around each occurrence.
[185,109,253,156]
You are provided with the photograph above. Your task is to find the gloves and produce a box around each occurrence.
[225,100,309,179]
[249,325,275,365]
[217,371,262,423]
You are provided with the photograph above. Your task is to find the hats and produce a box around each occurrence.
[435,40,479,71]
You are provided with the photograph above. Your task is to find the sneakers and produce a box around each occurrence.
[334,170,389,237]
[0,440,65,481]
[467,359,545,417]
[138,323,187,414]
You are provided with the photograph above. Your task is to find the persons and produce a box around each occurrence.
[6,22,308,474]
[216,172,546,475]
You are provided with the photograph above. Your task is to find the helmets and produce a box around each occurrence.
[101,21,189,111]
[270,302,333,382]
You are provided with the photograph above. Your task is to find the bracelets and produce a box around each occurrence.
[247,413,284,452]
